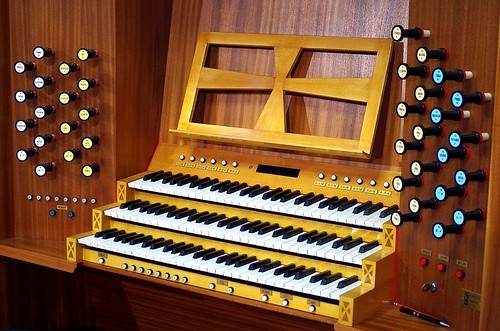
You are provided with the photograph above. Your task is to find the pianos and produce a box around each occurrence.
[65,140,397,327]
